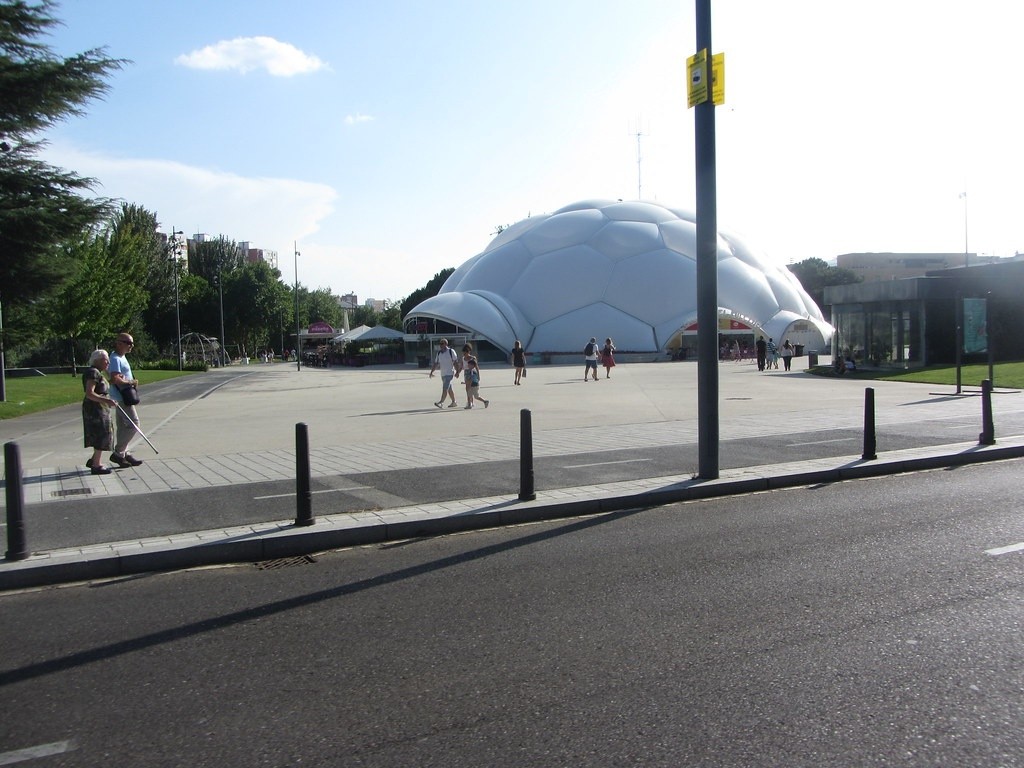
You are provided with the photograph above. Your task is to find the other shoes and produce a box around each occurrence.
[514,380,521,385]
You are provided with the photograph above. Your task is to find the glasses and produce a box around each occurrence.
[117,340,134,345]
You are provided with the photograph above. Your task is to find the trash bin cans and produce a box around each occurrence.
[416,355,427,368]
[794,344,805,357]
[808,350,819,369]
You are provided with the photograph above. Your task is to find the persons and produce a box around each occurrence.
[285,349,289,361]
[733,341,741,361]
[107,334,144,469]
[781,339,794,371]
[317,346,327,367]
[720,346,726,362]
[429,339,459,408]
[81,349,115,474]
[291,349,296,359]
[584,337,601,381]
[601,338,616,378]
[766,337,775,369]
[756,336,766,371]
[262,349,267,362]
[456,343,489,409]
[512,341,526,385]
[773,347,779,370]
[269,349,274,362]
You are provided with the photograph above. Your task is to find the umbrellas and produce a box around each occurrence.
[334,323,404,352]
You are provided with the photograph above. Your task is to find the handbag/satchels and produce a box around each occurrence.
[121,387,141,405]
[522,368,527,378]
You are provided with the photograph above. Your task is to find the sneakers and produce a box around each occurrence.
[464,404,473,409]
[485,400,490,408]
[435,402,444,409]
[86,459,112,475]
[110,453,143,467]
[448,401,458,408]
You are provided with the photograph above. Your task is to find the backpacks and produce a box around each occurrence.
[604,347,612,356]
[584,343,595,356]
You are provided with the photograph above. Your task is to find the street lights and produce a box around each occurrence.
[295,251,300,371]
[166,231,188,371]
